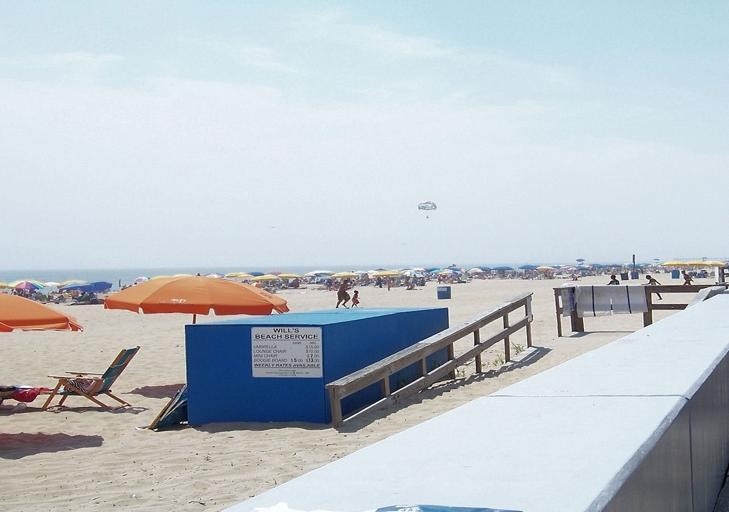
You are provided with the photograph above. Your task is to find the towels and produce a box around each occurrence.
[562,284,648,319]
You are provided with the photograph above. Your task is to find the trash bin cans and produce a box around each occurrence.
[437,286,451,299]
[672,270,679,279]
[631,272,638,279]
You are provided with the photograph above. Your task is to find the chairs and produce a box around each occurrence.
[0,346,141,411]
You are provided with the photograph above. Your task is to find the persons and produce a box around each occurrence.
[352,290,361,307]
[641,275,662,300]
[335,280,352,308]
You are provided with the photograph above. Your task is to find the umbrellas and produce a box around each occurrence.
[0,292,85,332]
[103,275,289,324]
[0,279,112,306]
